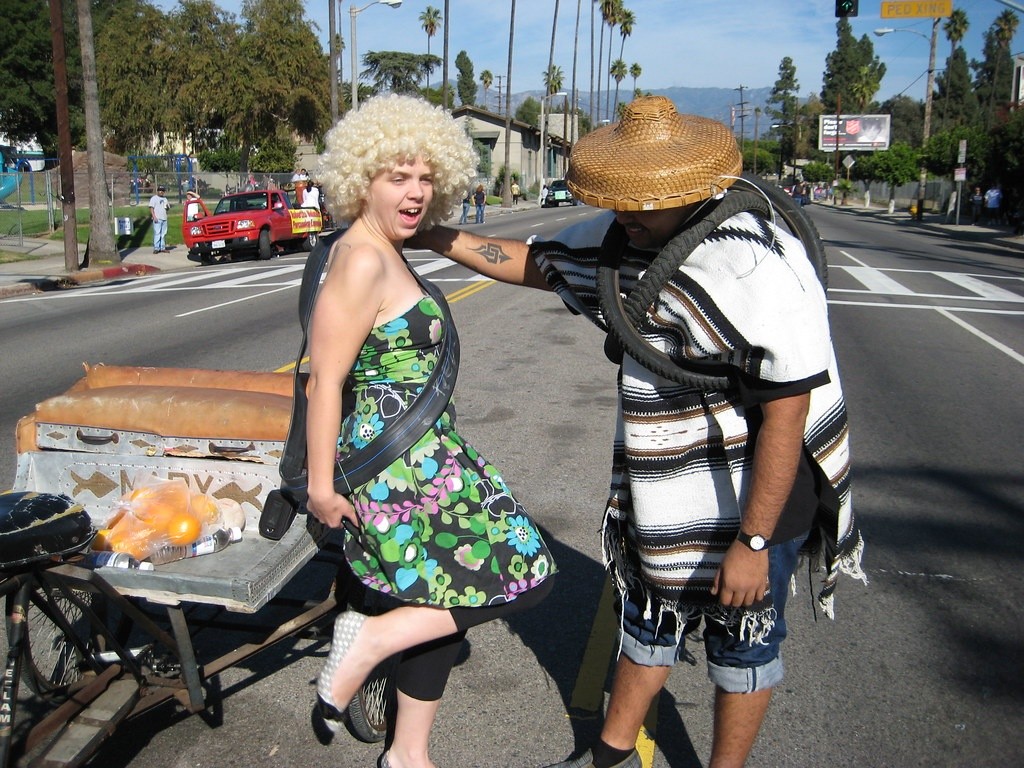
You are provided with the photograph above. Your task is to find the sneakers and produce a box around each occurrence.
[541,747,643,768]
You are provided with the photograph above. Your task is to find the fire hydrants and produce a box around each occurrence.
[908,205,917,221]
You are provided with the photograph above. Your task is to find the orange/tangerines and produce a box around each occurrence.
[92,487,215,562]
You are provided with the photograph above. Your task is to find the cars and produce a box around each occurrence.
[544,180,582,207]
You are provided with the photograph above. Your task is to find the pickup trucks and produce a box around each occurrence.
[180,189,323,264]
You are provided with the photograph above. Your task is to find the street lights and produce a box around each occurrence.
[873,27,938,222]
[539,91,568,195]
[349,0,402,113]
[771,124,797,185]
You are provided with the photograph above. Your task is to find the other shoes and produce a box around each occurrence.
[380,750,440,768]
[972,223,975,225]
[315,610,368,734]
[188,250,198,256]
[160,250,170,253]
[153,250,160,254]
[481,221,485,224]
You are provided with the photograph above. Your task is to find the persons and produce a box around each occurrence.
[184,189,200,253]
[969,180,1014,227]
[149,186,171,254]
[306,95,559,768]
[405,97,867,768]
[291,168,320,212]
[458,184,486,225]
[511,181,520,205]
[244,173,275,193]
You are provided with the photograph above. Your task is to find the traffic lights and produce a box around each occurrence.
[835,0,858,17]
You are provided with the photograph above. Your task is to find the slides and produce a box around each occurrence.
[0,151,23,204]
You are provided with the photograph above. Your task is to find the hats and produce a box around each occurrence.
[566,96,743,212]
[157,185,166,191]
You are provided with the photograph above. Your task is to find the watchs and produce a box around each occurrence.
[737,529,770,553]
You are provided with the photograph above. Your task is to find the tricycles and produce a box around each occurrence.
[0,362,397,768]
